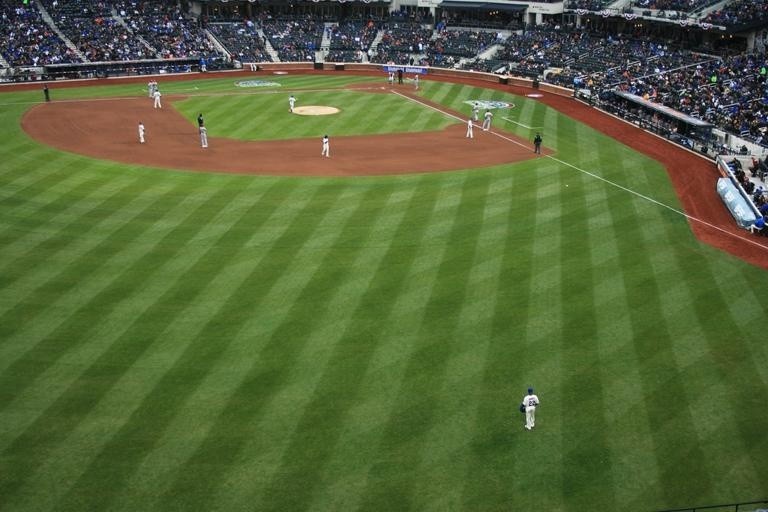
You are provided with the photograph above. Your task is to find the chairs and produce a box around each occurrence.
[0,1,574,86]
[575,0,768,162]
[716,154,768,231]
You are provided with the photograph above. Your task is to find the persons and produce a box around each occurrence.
[138,121,146,144]
[198,114,204,134]
[519,387,539,430]
[472,103,478,121]
[533,132,541,155]
[466,118,473,138]
[481,109,493,131]
[321,135,330,157]
[561,84,767,238]
[42,84,53,102]
[147,80,153,98]
[200,123,209,148]
[289,95,297,112]
[152,90,162,109]
